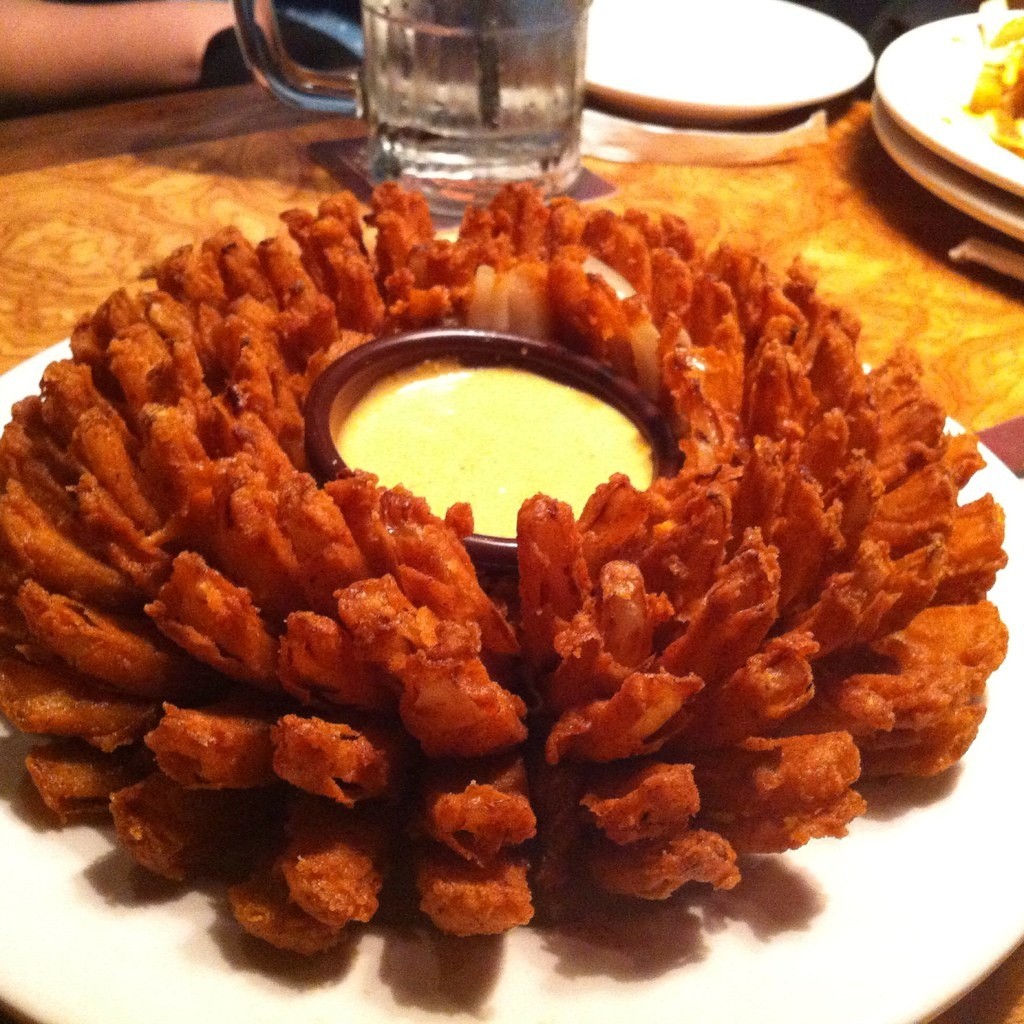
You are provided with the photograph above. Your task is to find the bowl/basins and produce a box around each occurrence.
[301,329,683,568]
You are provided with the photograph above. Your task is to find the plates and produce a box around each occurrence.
[871,89,1024,243]
[586,0,876,132]
[873,10,1024,202]
[0,336,1024,1024]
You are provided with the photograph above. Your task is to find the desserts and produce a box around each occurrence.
[330,363,658,539]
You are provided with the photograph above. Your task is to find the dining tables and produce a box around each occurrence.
[0,80,1024,1024]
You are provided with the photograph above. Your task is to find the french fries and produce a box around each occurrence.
[968,0,1024,154]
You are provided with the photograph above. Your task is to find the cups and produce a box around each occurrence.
[228,0,594,217]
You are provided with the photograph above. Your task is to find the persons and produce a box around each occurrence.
[0,0,277,109]
